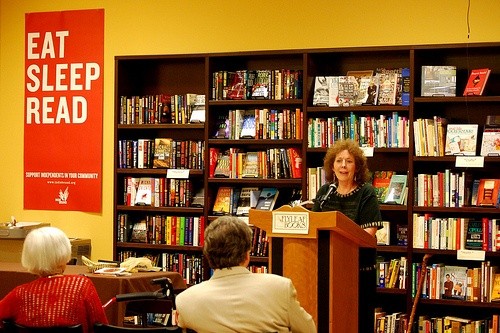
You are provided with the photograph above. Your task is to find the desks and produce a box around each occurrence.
[0,262,188,327]
[0,227,34,265]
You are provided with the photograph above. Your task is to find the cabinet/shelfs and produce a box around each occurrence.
[113,42,500,333]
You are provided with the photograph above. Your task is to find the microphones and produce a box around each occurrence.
[320,182,338,209]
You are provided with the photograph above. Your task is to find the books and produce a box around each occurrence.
[119,137,202,169]
[209,147,300,179]
[375,220,407,244]
[116,213,201,246]
[212,69,302,100]
[413,115,500,253]
[119,93,205,124]
[207,186,303,273]
[118,250,202,284]
[371,257,500,333]
[307,165,408,205]
[122,174,191,207]
[420,65,491,96]
[126,311,171,325]
[311,69,410,106]
[308,112,409,148]
[212,107,304,139]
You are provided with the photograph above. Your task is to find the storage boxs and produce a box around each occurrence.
[66,239,92,265]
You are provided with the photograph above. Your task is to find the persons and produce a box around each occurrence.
[174,216,316,333]
[311,137,384,333]
[0,226,109,333]
